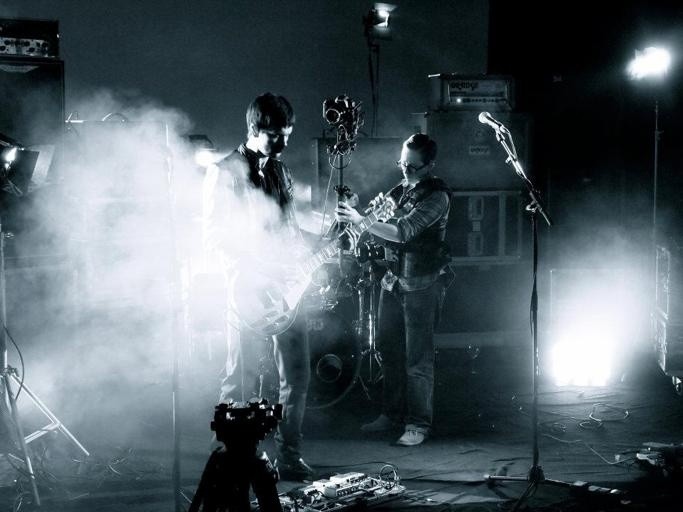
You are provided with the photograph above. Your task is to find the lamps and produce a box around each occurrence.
[1,143,56,197]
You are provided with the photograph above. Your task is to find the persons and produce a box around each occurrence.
[209,91,360,478]
[335,132,452,447]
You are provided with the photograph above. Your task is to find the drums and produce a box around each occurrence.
[302,251,361,300]
[252,304,362,411]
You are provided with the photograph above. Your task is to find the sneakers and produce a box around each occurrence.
[272,457,316,475]
[395,423,430,445]
[360,413,393,433]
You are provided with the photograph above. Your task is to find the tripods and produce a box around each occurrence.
[489,133,571,511]
[0,228,90,506]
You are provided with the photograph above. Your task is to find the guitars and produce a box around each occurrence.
[228,185,405,337]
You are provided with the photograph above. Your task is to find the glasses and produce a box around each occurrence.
[396,161,427,174]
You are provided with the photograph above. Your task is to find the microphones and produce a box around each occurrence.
[478,111,508,134]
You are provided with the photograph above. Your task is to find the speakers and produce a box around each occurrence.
[309,135,404,211]
[0,57,65,145]
